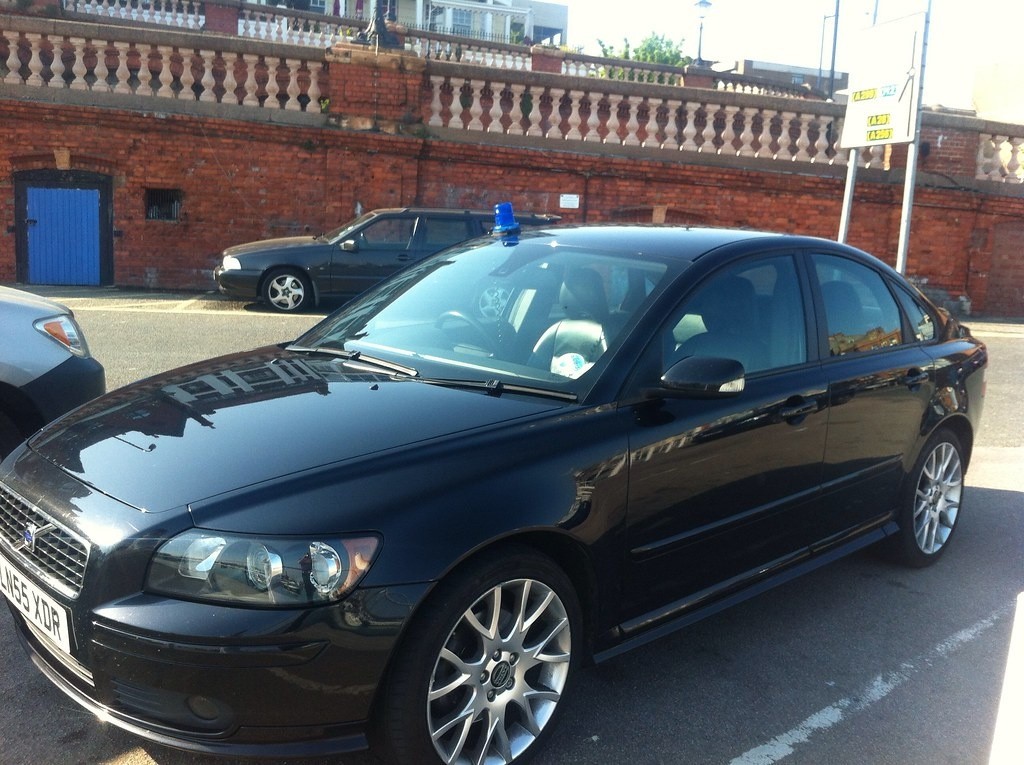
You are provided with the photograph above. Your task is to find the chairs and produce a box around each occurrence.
[664,275,771,378]
[525,268,617,376]
[818,281,863,341]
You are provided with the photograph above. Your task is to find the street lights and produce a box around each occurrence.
[694,0,713,66]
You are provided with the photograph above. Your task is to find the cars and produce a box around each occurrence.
[910,280,973,318]
[0,224,986,764]
[0,284,107,464]
[213,206,562,320]
